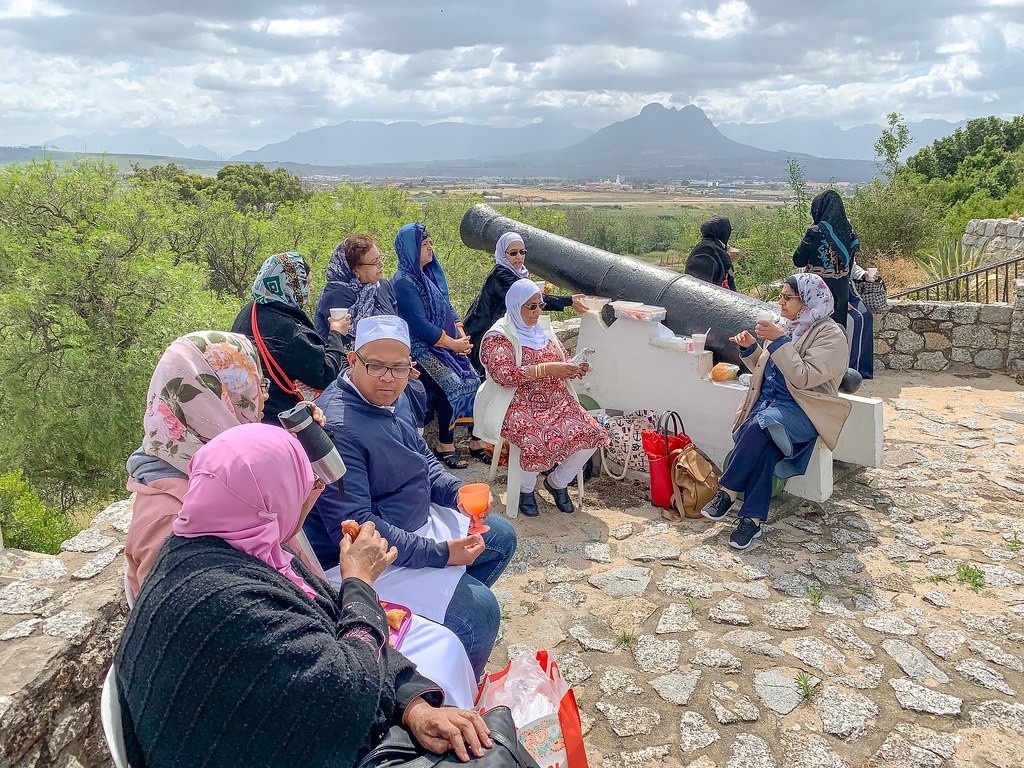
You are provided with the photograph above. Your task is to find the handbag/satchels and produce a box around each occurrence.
[356,706,540,768]
[642,409,692,506]
[853,275,889,311]
[474,650,588,767]
[600,410,661,480]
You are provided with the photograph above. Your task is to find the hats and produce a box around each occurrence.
[354,315,411,352]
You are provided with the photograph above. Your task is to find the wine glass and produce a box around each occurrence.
[458,483,491,534]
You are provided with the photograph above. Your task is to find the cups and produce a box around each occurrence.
[535,280,546,295]
[329,308,349,320]
[757,314,773,339]
[867,268,878,282]
[729,249,739,262]
[691,334,707,353]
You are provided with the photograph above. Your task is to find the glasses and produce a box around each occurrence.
[505,249,527,256]
[259,378,271,393]
[352,255,385,267]
[524,302,547,310]
[311,473,324,492]
[355,352,412,379]
[780,293,801,301]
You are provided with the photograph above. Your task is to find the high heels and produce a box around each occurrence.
[433,447,468,469]
[469,446,493,465]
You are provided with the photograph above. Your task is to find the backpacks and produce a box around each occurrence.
[661,443,722,523]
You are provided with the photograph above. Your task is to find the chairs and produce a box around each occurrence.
[491,436,585,517]
[101,664,131,768]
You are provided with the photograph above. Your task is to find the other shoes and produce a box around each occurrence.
[519,491,539,517]
[543,477,574,513]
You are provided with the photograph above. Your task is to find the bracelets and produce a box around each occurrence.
[535,361,550,379]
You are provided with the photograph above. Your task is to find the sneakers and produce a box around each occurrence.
[701,490,736,521]
[728,517,761,549]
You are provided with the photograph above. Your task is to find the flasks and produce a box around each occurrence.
[278,404,347,484]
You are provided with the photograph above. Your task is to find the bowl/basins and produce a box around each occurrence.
[581,296,612,311]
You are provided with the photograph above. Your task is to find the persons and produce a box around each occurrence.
[684,216,739,291]
[389,222,493,470]
[479,278,608,517]
[461,231,590,379]
[700,273,848,551]
[314,233,427,436]
[793,190,880,379]
[115,315,519,768]
[230,252,351,430]
